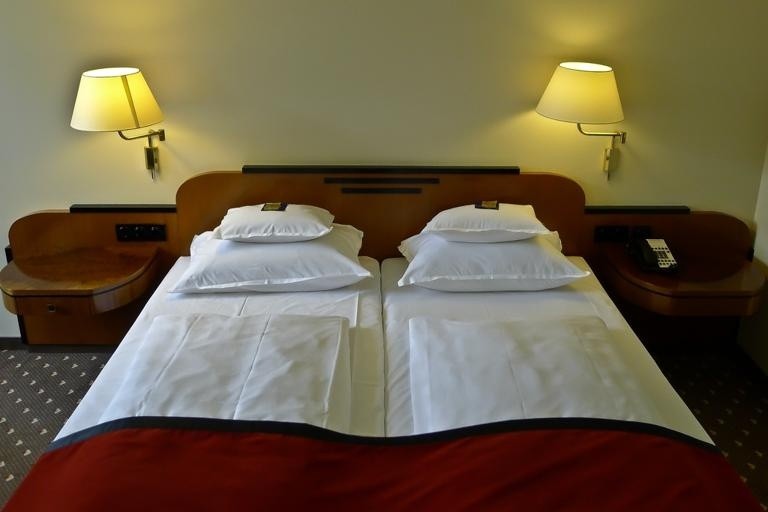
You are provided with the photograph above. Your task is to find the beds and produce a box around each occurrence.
[4,172,761,511]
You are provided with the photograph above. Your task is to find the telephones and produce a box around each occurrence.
[633,238,680,279]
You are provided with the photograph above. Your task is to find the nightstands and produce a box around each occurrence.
[0,248,158,316]
[600,237,767,315]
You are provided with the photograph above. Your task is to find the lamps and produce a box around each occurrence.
[536,63,626,182]
[70,66,166,181]
